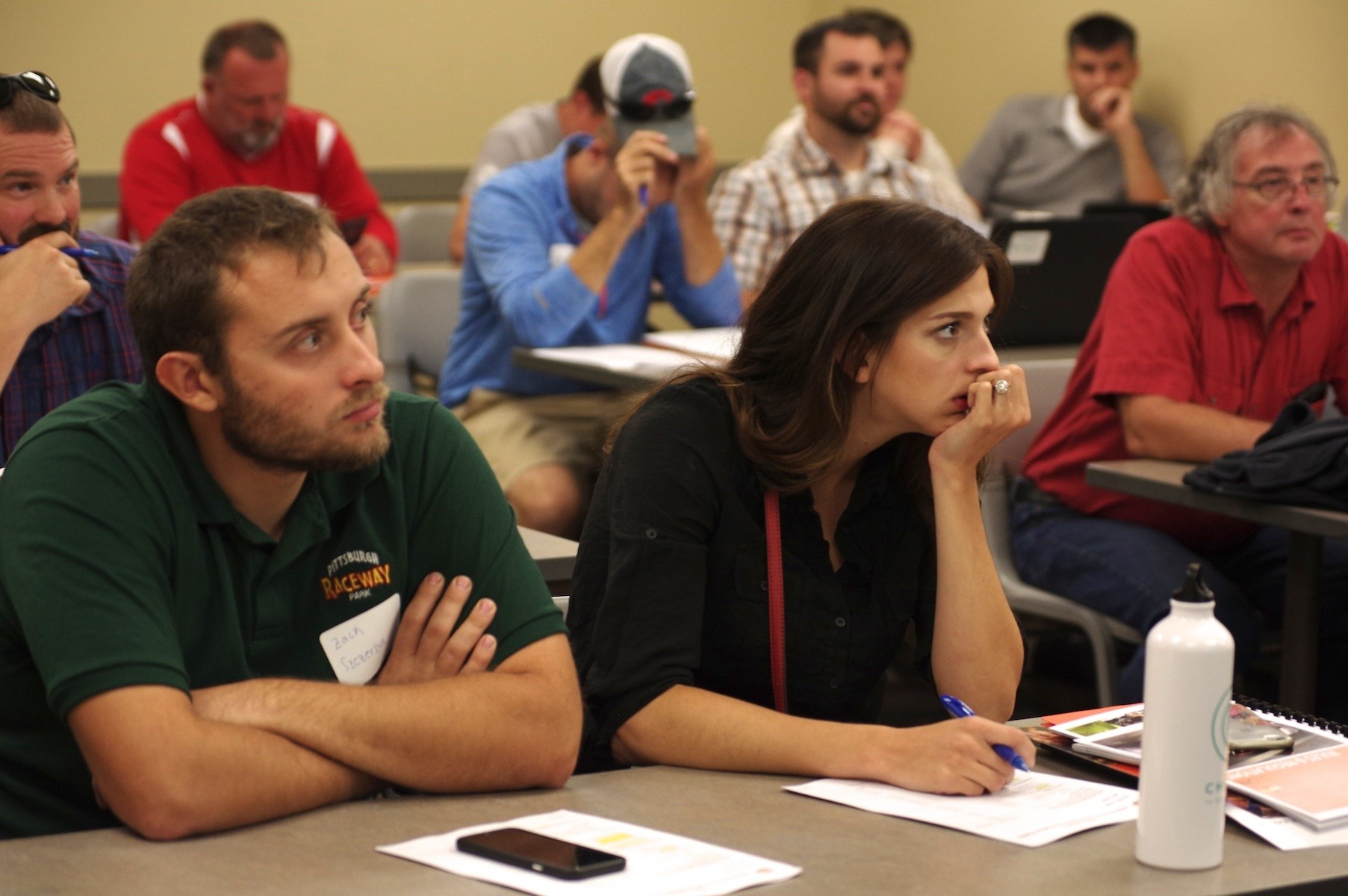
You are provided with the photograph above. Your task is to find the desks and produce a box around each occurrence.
[1086,451,1348,714]
[0,707,1348,896]
[512,331,1082,390]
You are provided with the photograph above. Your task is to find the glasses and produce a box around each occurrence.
[603,91,694,123]
[0,71,61,109]
[1227,173,1338,201]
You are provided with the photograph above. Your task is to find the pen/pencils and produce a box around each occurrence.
[0,243,99,258]
[639,185,647,206]
[941,694,1032,770]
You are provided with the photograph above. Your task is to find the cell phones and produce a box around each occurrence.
[1229,703,1294,752]
[456,828,626,879]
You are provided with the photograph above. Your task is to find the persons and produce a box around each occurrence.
[0,71,146,476]
[0,190,582,844]
[437,36,744,596]
[566,198,1036,796]
[119,18,399,279]
[699,9,983,326]
[1005,114,1348,710]
[956,11,1186,226]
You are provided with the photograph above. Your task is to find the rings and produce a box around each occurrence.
[993,380,1011,395]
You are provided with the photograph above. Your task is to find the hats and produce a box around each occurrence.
[599,33,698,159]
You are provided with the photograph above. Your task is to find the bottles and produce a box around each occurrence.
[1133,562,1235,872]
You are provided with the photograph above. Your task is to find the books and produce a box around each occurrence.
[1049,700,1348,831]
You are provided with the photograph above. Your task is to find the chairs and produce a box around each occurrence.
[397,204,455,260]
[976,362,1146,716]
[377,270,463,398]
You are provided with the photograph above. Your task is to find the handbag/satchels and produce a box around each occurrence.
[1184,384,1348,514]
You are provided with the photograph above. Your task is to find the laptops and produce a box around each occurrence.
[981,201,1170,349]
